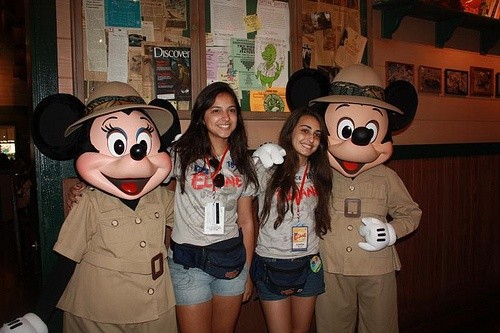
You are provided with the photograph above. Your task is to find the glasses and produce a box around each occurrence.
[209,156,226,189]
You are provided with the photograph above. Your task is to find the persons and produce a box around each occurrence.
[242,109,333,333]
[68,82,259,333]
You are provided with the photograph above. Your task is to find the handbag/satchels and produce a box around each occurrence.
[173,228,246,280]
[259,260,309,296]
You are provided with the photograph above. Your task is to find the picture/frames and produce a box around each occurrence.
[444,68,469,96]
[385,61,416,89]
[469,66,495,98]
[417,64,443,95]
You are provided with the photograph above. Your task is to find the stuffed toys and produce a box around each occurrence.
[32,81,175,333]
[251,64,422,333]
